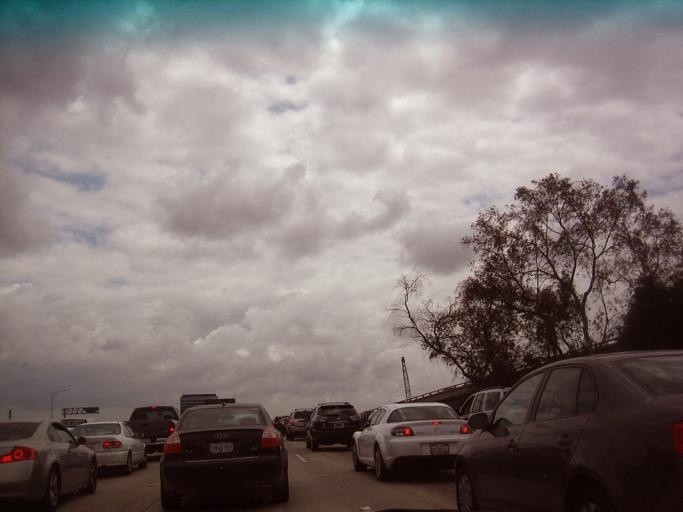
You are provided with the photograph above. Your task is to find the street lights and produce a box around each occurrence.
[51,388,69,422]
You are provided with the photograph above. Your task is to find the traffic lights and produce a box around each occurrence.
[62,408,101,416]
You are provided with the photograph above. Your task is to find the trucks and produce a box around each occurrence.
[180,395,234,415]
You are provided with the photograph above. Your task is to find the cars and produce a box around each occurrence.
[274,415,289,433]
[459,388,513,424]
[161,403,287,511]
[61,419,96,436]
[0,420,98,511]
[287,409,312,440]
[455,351,682,512]
[354,402,468,478]
[71,422,147,473]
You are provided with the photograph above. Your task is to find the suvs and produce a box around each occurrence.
[123,406,181,456]
[308,402,362,450]
